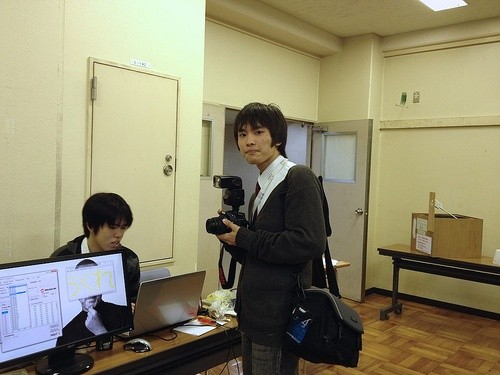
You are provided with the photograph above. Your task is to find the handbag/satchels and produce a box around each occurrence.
[281,287,363,369]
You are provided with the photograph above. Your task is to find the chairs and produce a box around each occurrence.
[139,267,170,285]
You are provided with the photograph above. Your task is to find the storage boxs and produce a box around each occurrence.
[410,192,484,257]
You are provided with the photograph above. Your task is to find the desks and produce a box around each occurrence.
[377,243,500,321]
[0,305,242,375]
[323,257,351,275]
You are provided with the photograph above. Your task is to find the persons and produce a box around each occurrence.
[215,102,327,375]
[55,259,131,348]
[50,191,141,329]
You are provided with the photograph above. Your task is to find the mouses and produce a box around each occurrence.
[124,339,153,353]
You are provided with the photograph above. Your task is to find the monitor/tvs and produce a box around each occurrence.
[0,248,135,375]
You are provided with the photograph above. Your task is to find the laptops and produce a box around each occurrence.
[117,270,207,338]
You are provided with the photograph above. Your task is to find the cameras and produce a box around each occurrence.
[205,175,247,234]
